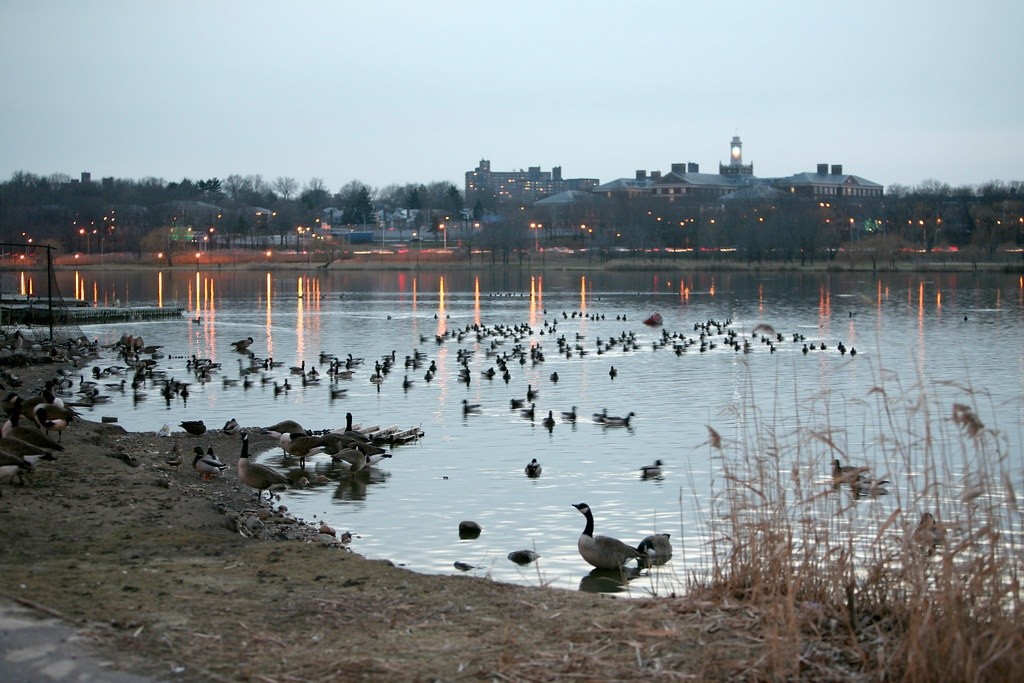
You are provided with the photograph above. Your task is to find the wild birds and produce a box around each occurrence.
[0,290,970,572]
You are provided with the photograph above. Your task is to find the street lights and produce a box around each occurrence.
[298,226,311,254]
[79,228,98,254]
[582,224,592,247]
[24,238,33,259]
[530,222,543,252]
[438,223,448,252]
